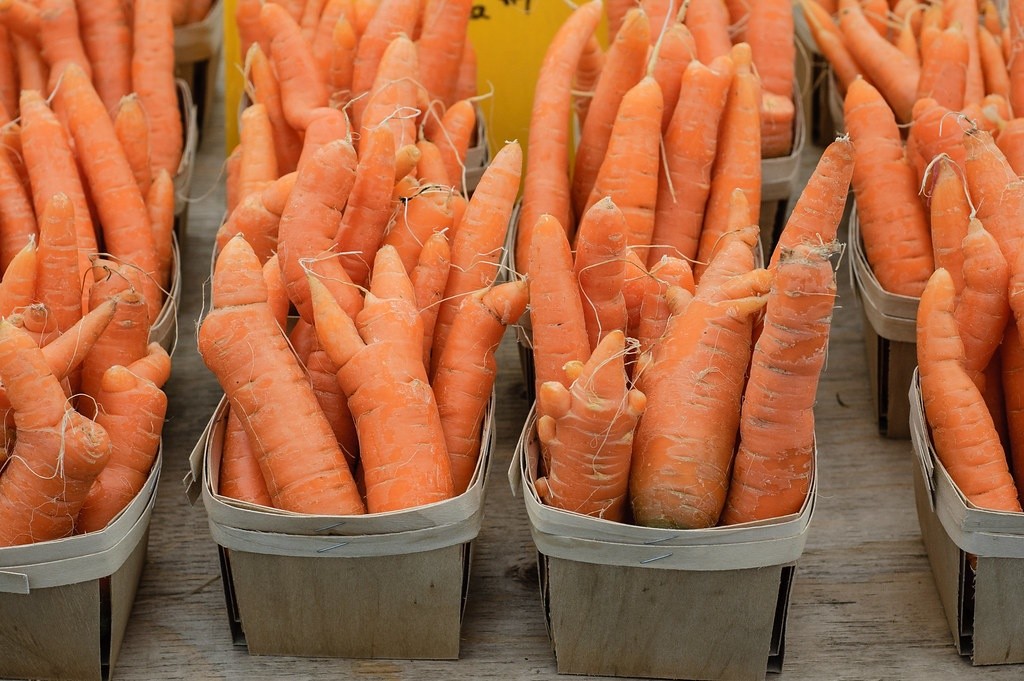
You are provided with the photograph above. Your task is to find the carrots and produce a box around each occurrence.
[518,0,1024,528]
[0,0,219,548]
[195,0,530,517]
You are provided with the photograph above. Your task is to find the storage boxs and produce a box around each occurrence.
[0,22,496,681]
[507,44,1024,681]
